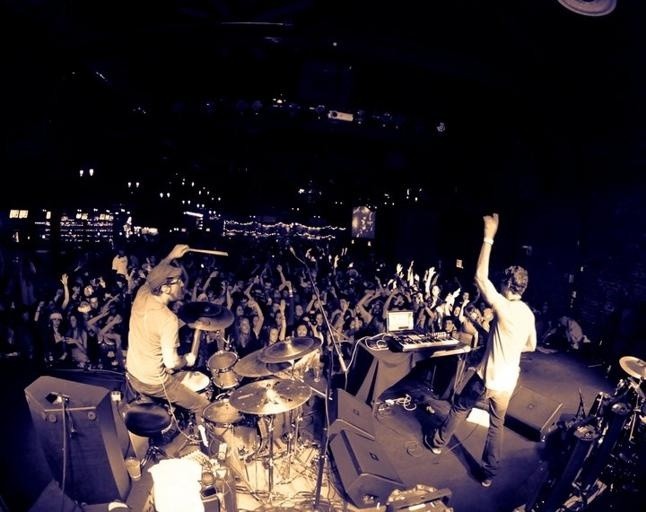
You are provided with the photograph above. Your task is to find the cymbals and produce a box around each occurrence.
[229,378,312,414]
[618,356,645,378]
[259,335,321,364]
[177,301,235,333]
[234,349,302,378]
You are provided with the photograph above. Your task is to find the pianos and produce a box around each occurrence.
[392,331,459,351]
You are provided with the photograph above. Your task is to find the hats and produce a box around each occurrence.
[146,263,183,289]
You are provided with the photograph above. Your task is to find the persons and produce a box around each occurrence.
[126,243,254,493]
[425,210,537,487]
[0,242,494,380]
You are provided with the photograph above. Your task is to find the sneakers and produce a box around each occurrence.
[425,433,443,455]
[480,467,493,488]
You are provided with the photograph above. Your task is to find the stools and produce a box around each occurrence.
[124,405,175,471]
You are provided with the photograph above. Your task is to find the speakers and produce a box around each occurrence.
[24,375,131,505]
[325,387,377,441]
[504,386,564,442]
[328,431,406,510]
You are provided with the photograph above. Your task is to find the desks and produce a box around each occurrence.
[357,342,473,418]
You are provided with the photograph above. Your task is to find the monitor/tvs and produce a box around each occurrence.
[387,311,415,334]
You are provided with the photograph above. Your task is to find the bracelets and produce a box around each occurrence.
[482,237,496,244]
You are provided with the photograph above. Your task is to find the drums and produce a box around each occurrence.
[207,350,245,389]
[202,399,267,459]
[169,370,213,401]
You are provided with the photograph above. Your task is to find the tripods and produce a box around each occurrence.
[237,361,309,512]
